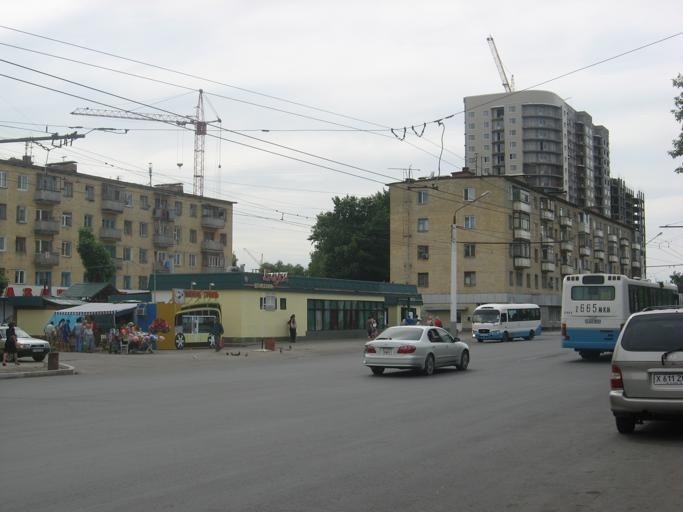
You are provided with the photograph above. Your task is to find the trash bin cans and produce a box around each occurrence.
[265,337,275,351]
[47,352,58,370]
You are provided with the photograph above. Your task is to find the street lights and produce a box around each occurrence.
[449,190,491,337]
[639,231,663,279]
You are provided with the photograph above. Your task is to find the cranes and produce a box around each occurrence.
[486,33,516,93]
[69,85,220,199]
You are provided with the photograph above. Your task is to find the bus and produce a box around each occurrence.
[557,272,679,361]
[470,303,543,342]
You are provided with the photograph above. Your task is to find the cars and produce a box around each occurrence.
[360,326,469,375]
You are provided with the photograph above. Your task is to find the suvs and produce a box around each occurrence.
[606,303,682,434]
[0,324,50,362]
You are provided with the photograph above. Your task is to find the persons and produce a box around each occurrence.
[2,321,20,368]
[364,315,379,341]
[401,314,442,329]
[45,316,157,353]
[288,313,297,342]
